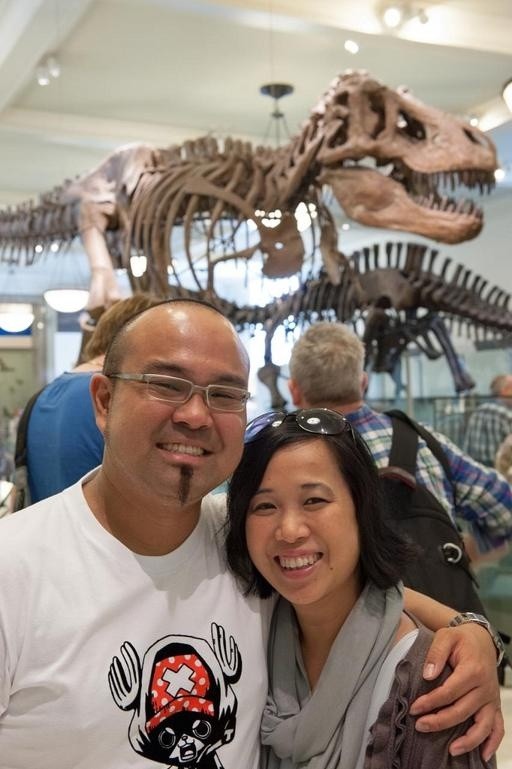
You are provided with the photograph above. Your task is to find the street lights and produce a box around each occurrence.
[374,466,506,686]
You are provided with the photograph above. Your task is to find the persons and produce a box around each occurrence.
[287,320,511,544]
[464,376,510,463]
[28,298,155,503]
[228,412,497,766]
[2,298,506,765]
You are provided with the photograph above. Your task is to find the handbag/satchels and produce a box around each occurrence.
[449,608,503,667]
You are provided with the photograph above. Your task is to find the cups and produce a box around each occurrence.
[242,408,357,451]
[106,372,251,412]
